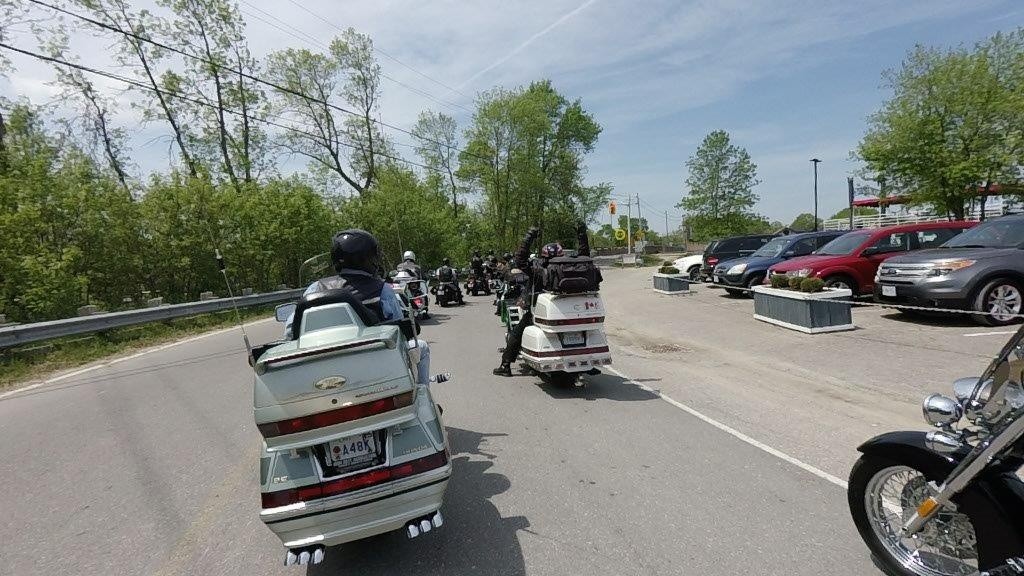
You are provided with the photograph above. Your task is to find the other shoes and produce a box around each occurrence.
[435,301,439,304]
[459,301,465,304]
[423,314,431,319]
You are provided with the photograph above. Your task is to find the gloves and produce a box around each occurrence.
[529,228,538,239]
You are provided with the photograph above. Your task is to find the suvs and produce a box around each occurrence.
[699,235,813,284]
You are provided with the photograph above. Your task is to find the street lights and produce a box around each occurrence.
[810,159,822,231]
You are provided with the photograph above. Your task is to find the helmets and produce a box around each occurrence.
[330,229,379,274]
[404,251,415,262]
[541,243,564,257]
[443,258,449,264]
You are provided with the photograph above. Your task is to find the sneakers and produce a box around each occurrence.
[493,364,511,376]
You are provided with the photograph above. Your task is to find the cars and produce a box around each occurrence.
[763,221,1001,299]
[710,230,856,297]
[872,211,1024,326]
[672,254,709,284]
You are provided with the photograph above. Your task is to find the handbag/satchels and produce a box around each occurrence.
[542,255,603,294]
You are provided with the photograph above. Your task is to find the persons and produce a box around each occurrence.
[435,258,466,305]
[493,218,591,377]
[397,250,431,320]
[467,248,537,316]
[285,229,443,417]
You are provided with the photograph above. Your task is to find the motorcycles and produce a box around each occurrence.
[430,267,459,307]
[250,251,453,564]
[846,317,1024,576]
[466,253,613,389]
[389,269,428,320]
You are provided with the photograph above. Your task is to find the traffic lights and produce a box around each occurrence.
[609,201,616,214]
